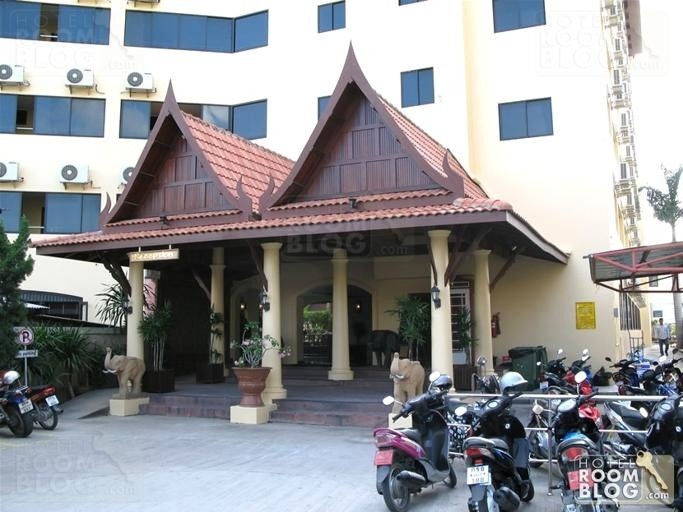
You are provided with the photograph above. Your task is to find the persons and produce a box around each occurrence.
[654,317,671,357]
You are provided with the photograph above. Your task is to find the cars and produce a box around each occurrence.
[460,347,683,512]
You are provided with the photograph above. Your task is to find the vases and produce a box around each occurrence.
[233,368,272,406]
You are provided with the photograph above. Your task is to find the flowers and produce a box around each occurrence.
[230,320,293,368]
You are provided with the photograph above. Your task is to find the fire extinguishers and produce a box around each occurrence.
[491,312,500,337]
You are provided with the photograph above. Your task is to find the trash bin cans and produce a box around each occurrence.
[508,346,547,389]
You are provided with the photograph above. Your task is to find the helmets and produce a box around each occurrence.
[3,370,21,385]
[434,376,452,390]
[500,371,529,395]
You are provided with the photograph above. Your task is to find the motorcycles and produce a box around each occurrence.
[21,379,64,431]
[0,368,37,439]
[372,370,459,512]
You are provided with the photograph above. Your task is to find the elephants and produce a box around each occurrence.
[102,347,146,394]
[389,352,425,413]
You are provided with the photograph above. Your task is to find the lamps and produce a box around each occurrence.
[431,286,441,308]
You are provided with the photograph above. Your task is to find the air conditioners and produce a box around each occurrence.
[125,72,154,90]
[0,162,19,181]
[0,63,25,84]
[60,163,89,183]
[63,68,94,88]
[120,165,136,184]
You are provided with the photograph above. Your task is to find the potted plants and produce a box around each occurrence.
[451,305,480,390]
[195,302,224,384]
[136,296,175,393]
